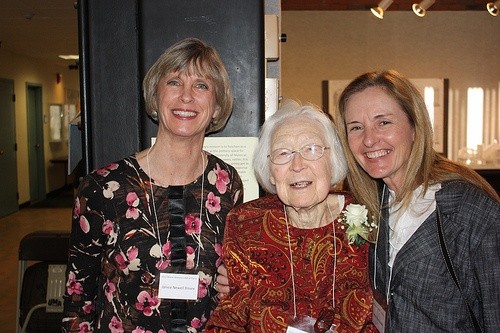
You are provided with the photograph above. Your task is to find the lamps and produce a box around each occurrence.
[412,0,435,17]
[486,0,500,16]
[370,0,393,19]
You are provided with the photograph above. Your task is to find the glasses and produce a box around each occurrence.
[266,144,331,165]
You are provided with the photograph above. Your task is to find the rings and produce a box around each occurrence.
[213,281,218,289]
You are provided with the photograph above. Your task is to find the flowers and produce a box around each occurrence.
[337,202,378,245]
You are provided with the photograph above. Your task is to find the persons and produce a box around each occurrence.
[202,98,378,333]
[214,69,500,333]
[61,38,244,333]
